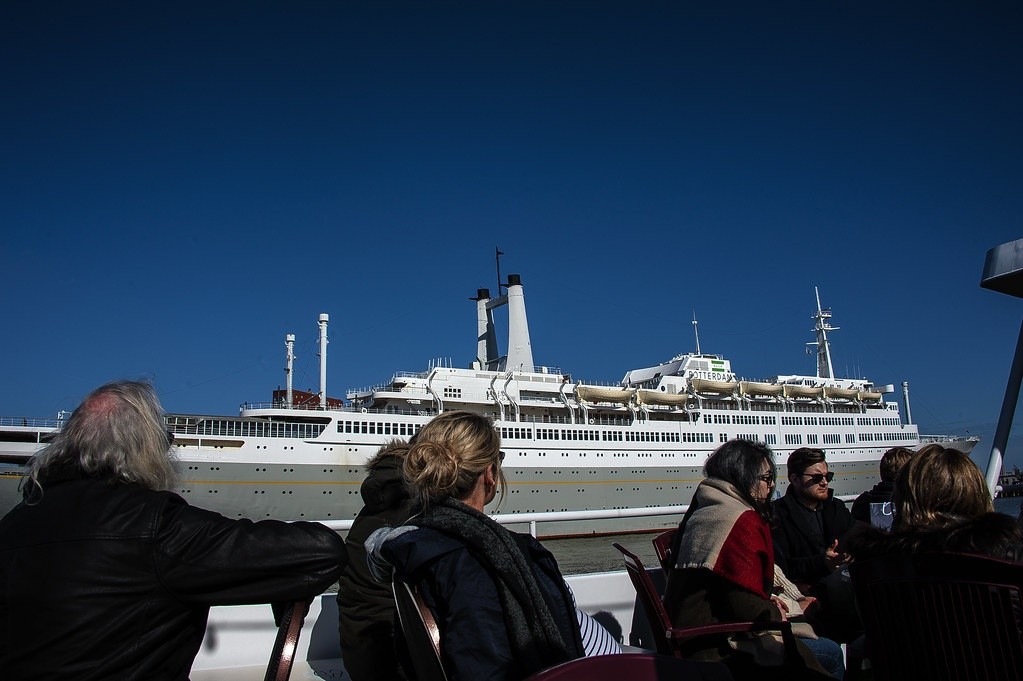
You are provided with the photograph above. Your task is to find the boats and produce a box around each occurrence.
[825,387,859,401]
[271,388,344,411]
[636,390,689,408]
[740,381,782,396]
[690,379,741,396]
[576,386,633,405]
[859,391,883,403]
[785,385,823,400]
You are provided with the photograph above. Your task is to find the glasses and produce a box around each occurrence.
[162,429,175,450]
[755,470,776,487]
[496,451,506,465]
[797,472,835,484]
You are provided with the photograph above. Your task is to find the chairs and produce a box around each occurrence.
[258,529,1023,681]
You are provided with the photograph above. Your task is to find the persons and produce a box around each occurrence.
[0,370,350,681]
[661,438,844,681]
[336,410,623,681]
[770,444,1023,681]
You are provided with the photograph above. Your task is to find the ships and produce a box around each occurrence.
[0,244,981,542]
